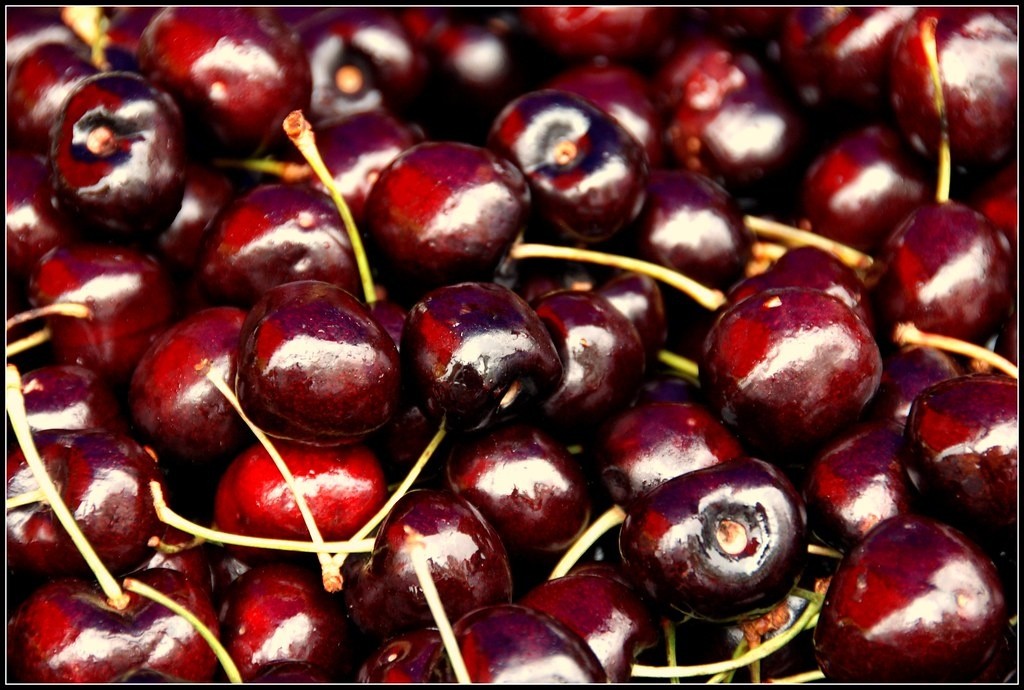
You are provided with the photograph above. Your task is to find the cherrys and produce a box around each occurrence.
[8,7,1017,684]
